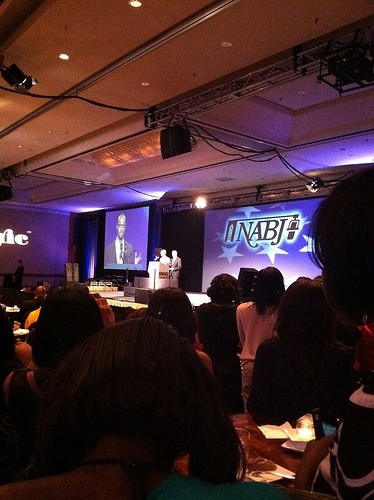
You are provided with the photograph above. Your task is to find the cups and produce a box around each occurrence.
[13,328,30,343]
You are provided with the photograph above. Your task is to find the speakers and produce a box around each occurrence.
[134,288,154,304]
[0,185,12,202]
[160,127,191,159]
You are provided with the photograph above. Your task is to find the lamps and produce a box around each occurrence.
[0,55,36,90]
[304,176,328,194]
[157,124,193,161]
[0,178,14,202]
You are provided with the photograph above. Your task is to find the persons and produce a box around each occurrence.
[14,260,25,288]
[0,316,293,500]
[146,287,212,376]
[159,249,171,266]
[0,289,106,477]
[193,274,244,413]
[244,277,355,424]
[104,213,142,264]
[169,250,182,289]
[0,285,115,486]
[235,268,284,413]
[296,165,374,500]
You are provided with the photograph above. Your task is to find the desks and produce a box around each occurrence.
[170,412,303,489]
